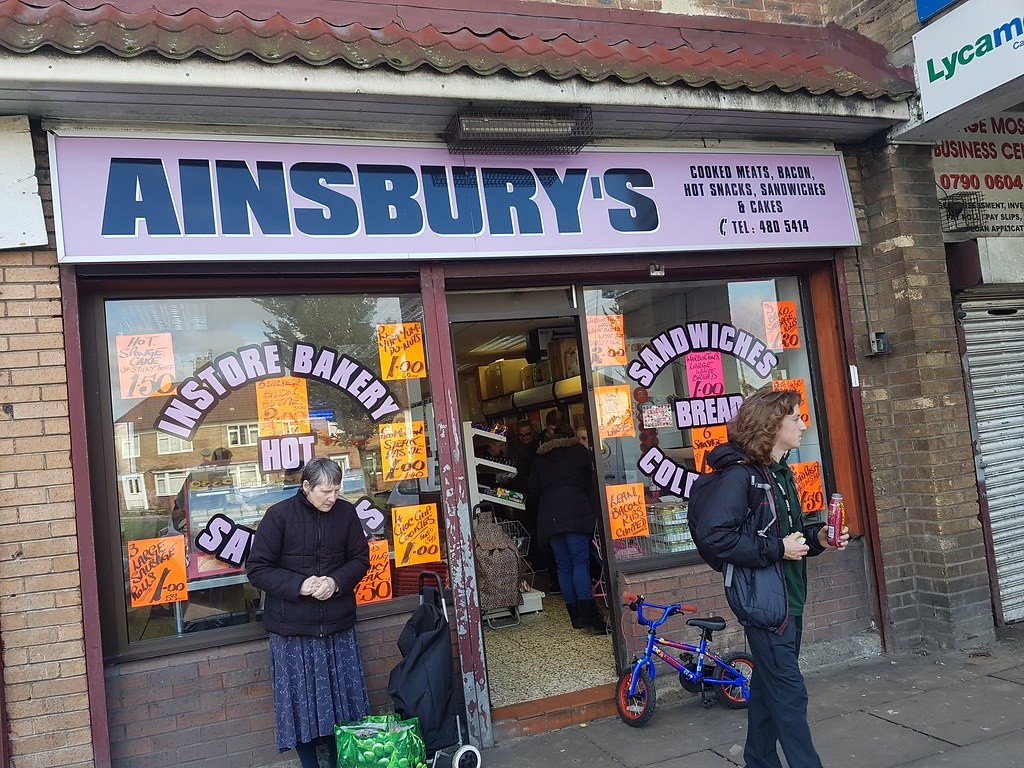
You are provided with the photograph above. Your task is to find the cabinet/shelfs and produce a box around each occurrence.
[463,420,547,620]
[645,502,696,554]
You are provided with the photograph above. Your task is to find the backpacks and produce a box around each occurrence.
[687,461,766,573]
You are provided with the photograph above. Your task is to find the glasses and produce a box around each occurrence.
[517,431,532,439]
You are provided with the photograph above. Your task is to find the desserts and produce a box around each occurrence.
[188,470,304,523]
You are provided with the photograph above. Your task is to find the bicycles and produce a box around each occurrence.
[615,591,755,727]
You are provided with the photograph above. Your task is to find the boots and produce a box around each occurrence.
[566,599,611,634]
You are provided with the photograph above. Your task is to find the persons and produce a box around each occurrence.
[698,387,849,768]
[171,447,236,535]
[478,410,613,633]
[245,456,371,768]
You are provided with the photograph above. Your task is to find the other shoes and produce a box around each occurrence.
[550,585,562,594]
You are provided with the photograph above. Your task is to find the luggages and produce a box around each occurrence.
[473,503,524,629]
[387,570,481,768]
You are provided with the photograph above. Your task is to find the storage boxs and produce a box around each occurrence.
[672,350,728,447]
[475,337,577,403]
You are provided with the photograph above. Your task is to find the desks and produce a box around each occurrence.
[171,540,395,637]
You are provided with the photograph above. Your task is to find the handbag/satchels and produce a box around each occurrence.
[334,714,427,768]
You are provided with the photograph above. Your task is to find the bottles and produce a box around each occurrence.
[827,493,845,547]
